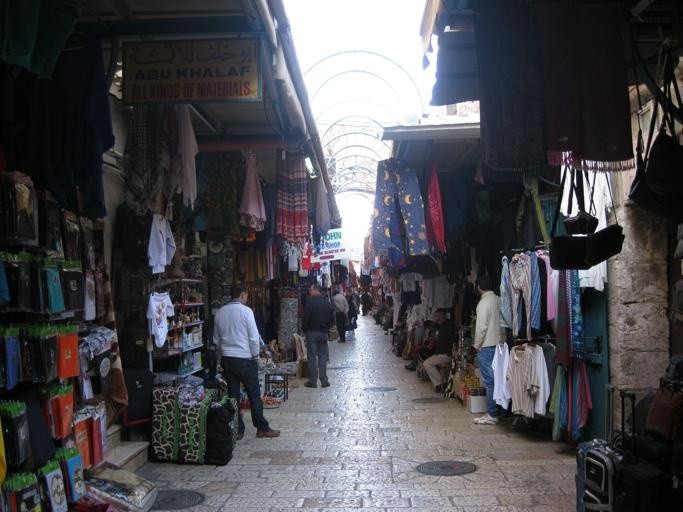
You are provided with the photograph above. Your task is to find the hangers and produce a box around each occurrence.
[498,243,549,265]
[498,333,552,353]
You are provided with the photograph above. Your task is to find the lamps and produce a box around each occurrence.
[277,143,288,161]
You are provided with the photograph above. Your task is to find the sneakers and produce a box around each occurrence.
[473,413,499,424]
[304,381,330,388]
[437,383,447,392]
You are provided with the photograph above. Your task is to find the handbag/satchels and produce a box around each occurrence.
[549,211,622,270]
[668,223,683,326]
[628,131,683,220]
[343,319,353,331]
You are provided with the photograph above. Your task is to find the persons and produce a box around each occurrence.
[471,276,503,425]
[403,319,434,371]
[210,285,281,439]
[421,307,458,392]
[300,283,385,388]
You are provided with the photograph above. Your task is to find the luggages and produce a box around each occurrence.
[576,355,683,511]
[151,381,238,466]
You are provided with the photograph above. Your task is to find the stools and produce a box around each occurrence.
[279,349,295,362]
[387,328,396,349]
[264,373,289,403]
[435,364,452,394]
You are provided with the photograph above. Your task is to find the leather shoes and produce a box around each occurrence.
[257,429,279,437]
[237,432,244,440]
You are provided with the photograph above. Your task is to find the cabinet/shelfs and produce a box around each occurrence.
[0,169,206,512]
